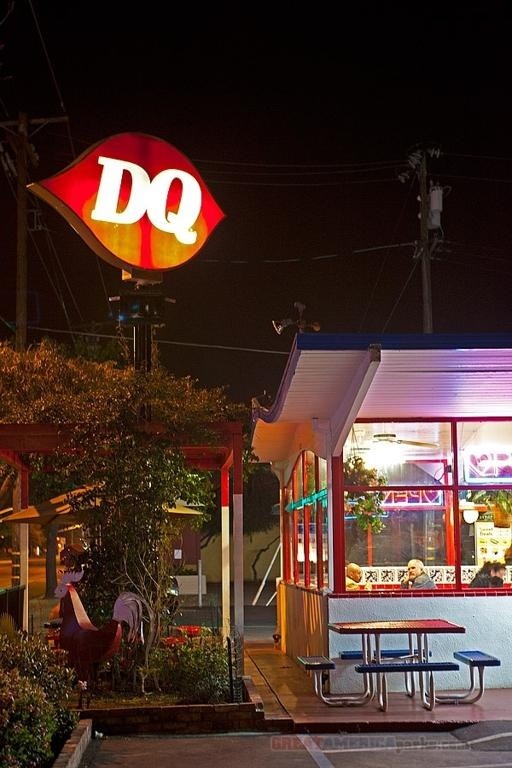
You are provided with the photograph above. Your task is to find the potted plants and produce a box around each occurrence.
[470,489,512,527]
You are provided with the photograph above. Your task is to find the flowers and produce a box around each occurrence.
[344,455,387,535]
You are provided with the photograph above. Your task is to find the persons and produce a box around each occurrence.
[468,561,506,589]
[345,562,372,591]
[401,558,438,589]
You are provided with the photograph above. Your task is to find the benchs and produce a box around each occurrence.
[453,651,501,704]
[339,649,433,697]
[297,655,336,706]
[355,661,459,712]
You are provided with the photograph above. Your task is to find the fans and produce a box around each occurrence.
[350,434,439,449]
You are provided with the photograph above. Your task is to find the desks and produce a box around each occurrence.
[328,618,465,711]
